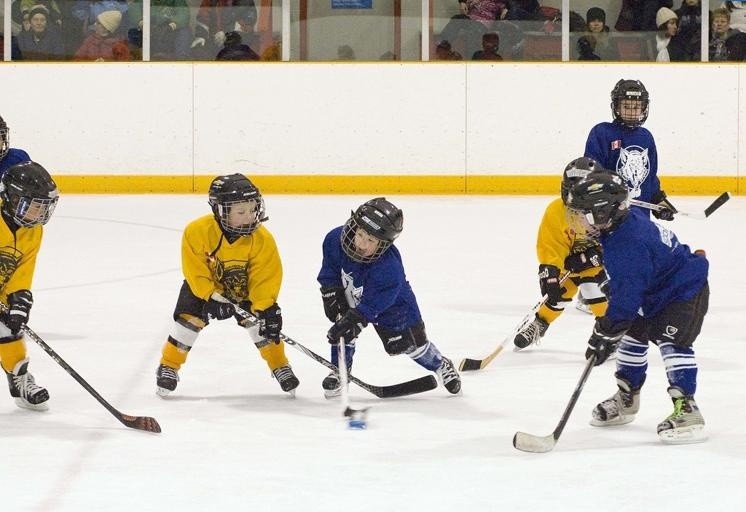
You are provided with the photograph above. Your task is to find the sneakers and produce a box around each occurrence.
[323,370,351,390]
[437,357,461,394]
[273,365,300,394]
[156,362,178,391]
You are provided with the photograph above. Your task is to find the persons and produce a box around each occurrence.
[0,0,262,62]
[318,198,463,399]
[0,162,60,411]
[1,117,31,179]
[157,174,300,400]
[693,9,746,61]
[585,77,675,221]
[438,1,540,62]
[581,171,710,442]
[512,156,617,360]
[573,0,711,62]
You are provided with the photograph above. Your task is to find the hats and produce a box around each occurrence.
[27,5,50,25]
[97,11,122,34]
[656,7,678,29]
[586,7,606,31]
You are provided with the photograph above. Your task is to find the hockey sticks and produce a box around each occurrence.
[211,292,437,399]
[457,272,572,372]
[629,191,730,221]
[336,311,370,417]
[0,302,161,432]
[512,353,596,452]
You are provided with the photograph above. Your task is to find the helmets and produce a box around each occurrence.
[0,161,60,228]
[340,197,402,262]
[208,174,268,239]
[561,157,607,216]
[0,116,10,158]
[564,169,631,241]
[611,79,649,131]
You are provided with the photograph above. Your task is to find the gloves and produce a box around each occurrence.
[5,289,34,340]
[597,272,611,302]
[327,308,367,346]
[258,302,283,344]
[206,290,252,320]
[564,248,604,274]
[191,38,205,49]
[585,317,632,365]
[320,284,349,324]
[538,263,567,306]
[650,190,677,221]
[214,30,226,47]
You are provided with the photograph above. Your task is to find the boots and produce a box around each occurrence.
[1,358,50,405]
[657,387,705,434]
[514,312,549,348]
[591,371,648,421]
